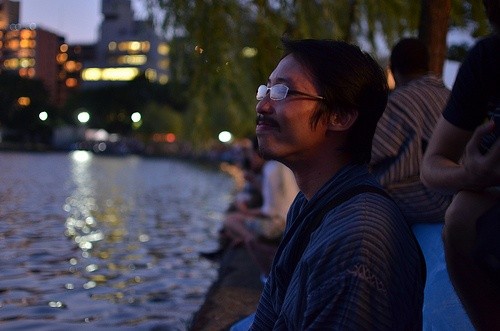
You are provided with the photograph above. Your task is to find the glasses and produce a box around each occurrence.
[256,84,326,101]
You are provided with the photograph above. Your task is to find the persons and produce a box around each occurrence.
[253,38,427,330]
[367,36,466,228]
[419,29,500,330]
[221,139,300,249]
